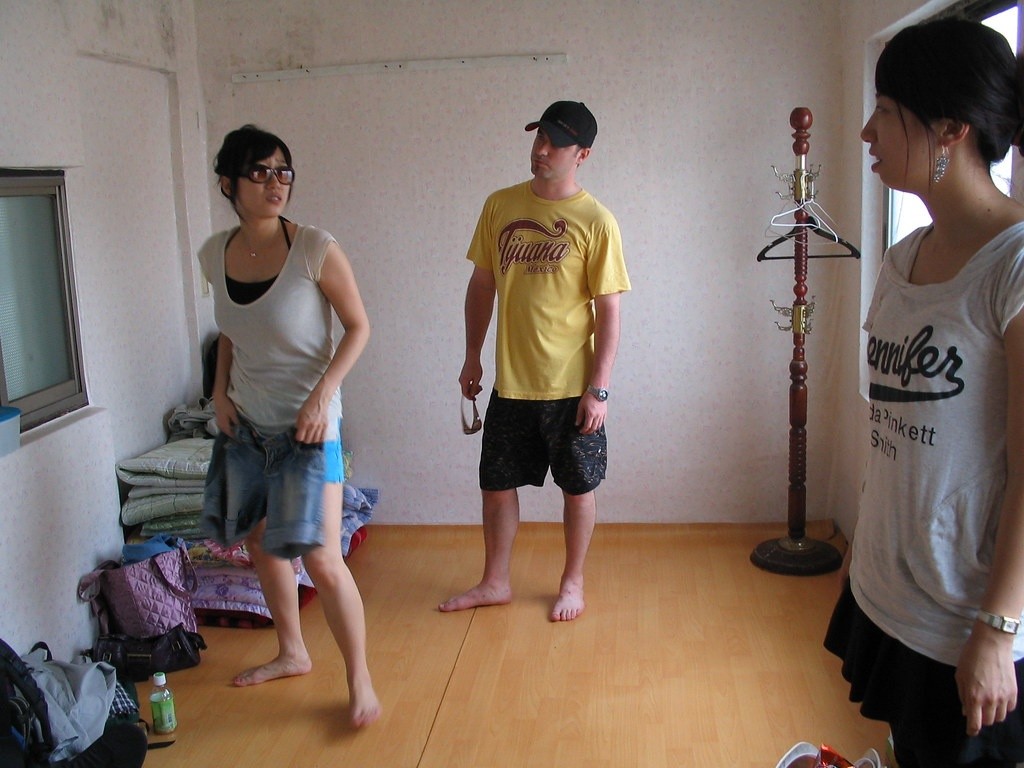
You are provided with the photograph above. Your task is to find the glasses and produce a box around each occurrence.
[233,165,295,185]
[460,393,481,435]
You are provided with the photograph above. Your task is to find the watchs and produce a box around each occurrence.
[976,609,1022,635]
[587,384,609,401]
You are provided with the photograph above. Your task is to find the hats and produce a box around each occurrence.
[525,101,598,151]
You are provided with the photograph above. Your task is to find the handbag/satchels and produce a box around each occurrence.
[94,539,199,636]
[88,631,206,679]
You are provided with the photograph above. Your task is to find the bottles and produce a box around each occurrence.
[149,672,178,735]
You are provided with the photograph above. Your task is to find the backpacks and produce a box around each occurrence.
[0,641,55,767]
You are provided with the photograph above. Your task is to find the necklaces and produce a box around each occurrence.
[248,247,258,257]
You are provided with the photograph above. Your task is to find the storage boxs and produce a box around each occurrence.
[0,406,22,458]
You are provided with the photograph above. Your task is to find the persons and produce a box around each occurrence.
[821,15,1024,768]
[195,126,384,730]
[436,101,631,620]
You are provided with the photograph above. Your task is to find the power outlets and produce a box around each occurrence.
[200,268,210,297]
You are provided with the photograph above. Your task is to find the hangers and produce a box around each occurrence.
[756,172,861,262]
